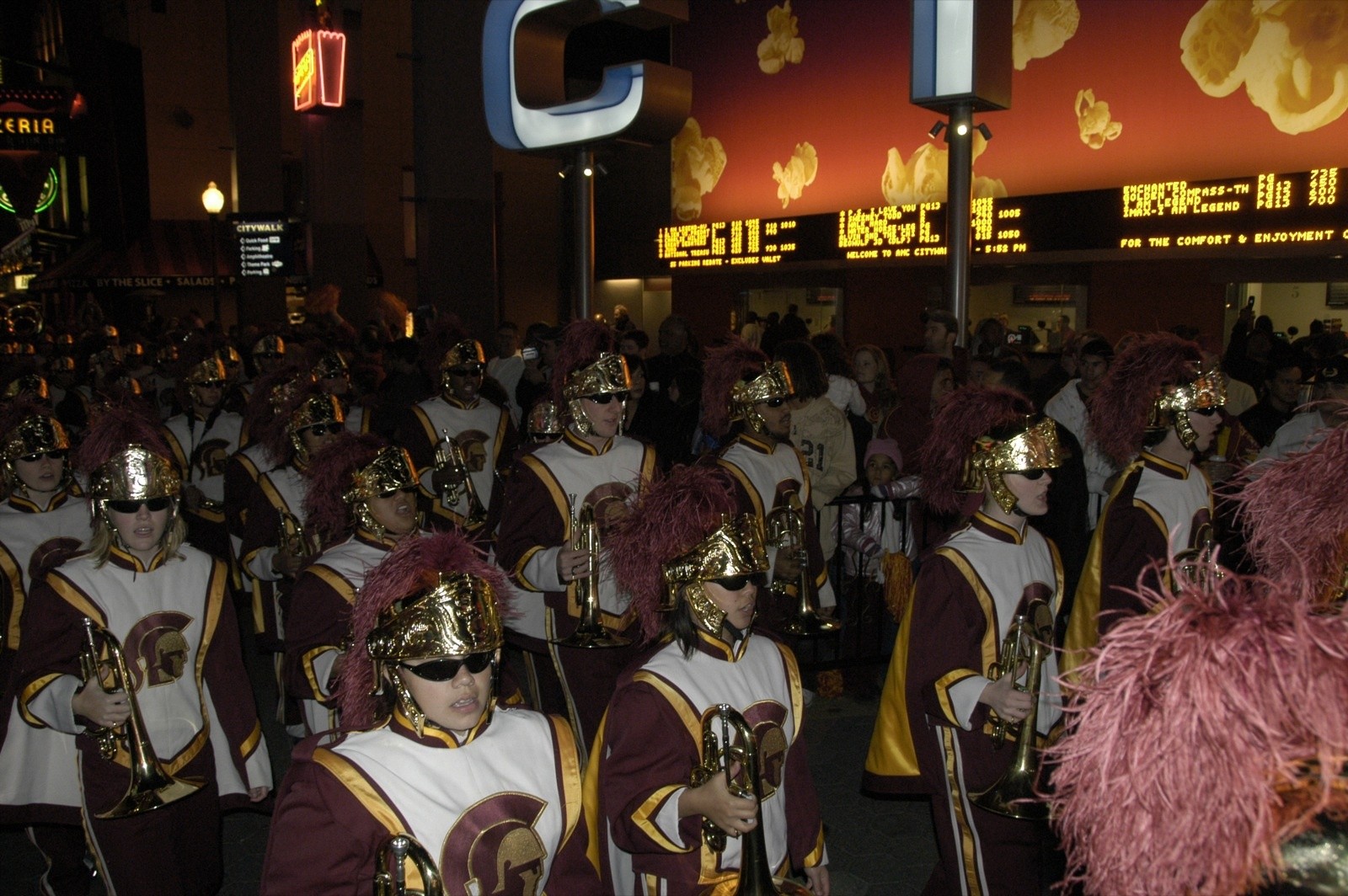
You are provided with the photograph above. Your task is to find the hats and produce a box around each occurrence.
[863,438,903,480]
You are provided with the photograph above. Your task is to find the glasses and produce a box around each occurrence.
[753,394,791,406]
[580,392,627,405]
[702,573,767,592]
[387,652,492,682]
[1002,469,1048,480]
[1191,406,1226,417]
[533,432,562,440]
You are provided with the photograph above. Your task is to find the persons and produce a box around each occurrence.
[7,296,1348,896]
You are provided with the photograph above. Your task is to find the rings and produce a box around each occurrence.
[572,576,574,580]
[572,566,578,575]
[735,829,738,838]
[1011,716,1014,725]
[113,721,117,728]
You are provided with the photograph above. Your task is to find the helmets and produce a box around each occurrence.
[1084,334,1229,467]
[922,384,1061,513]
[551,321,630,437]
[335,535,524,741]
[0,307,484,550]
[1045,412,1347,896]
[701,339,794,438]
[609,466,771,638]
[524,394,564,447]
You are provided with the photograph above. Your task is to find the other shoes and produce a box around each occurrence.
[802,688,815,708]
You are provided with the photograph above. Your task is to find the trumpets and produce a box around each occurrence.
[967,615,1061,821]
[765,505,846,636]
[690,703,816,896]
[373,834,444,896]
[1169,538,1224,595]
[276,506,312,580]
[433,428,489,528]
[77,617,209,821]
[548,493,636,650]
